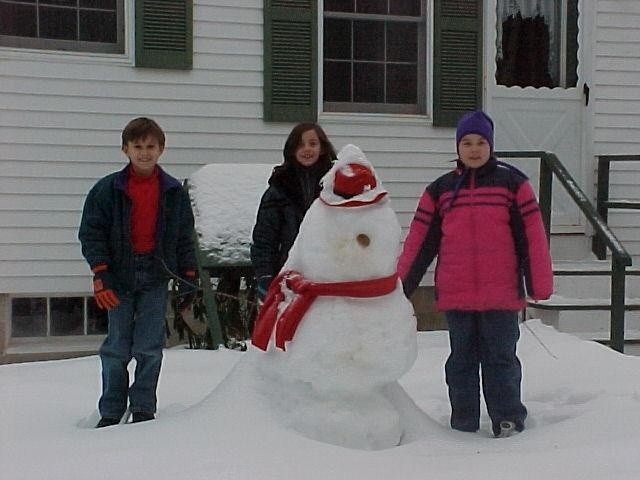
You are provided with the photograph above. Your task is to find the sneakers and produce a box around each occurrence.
[94,408,131,429]
[130,410,156,423]
[492,419,524,438]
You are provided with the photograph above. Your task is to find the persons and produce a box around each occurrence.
[249,122,336,295]
[395,109,554,438]
[76,117,200,429]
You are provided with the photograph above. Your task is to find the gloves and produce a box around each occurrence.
[178,271,196,314]
[256,276,274,307]
[91,264,121,310]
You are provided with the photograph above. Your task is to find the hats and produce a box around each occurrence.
[456,111,494,157]
[320,164,388,207]
